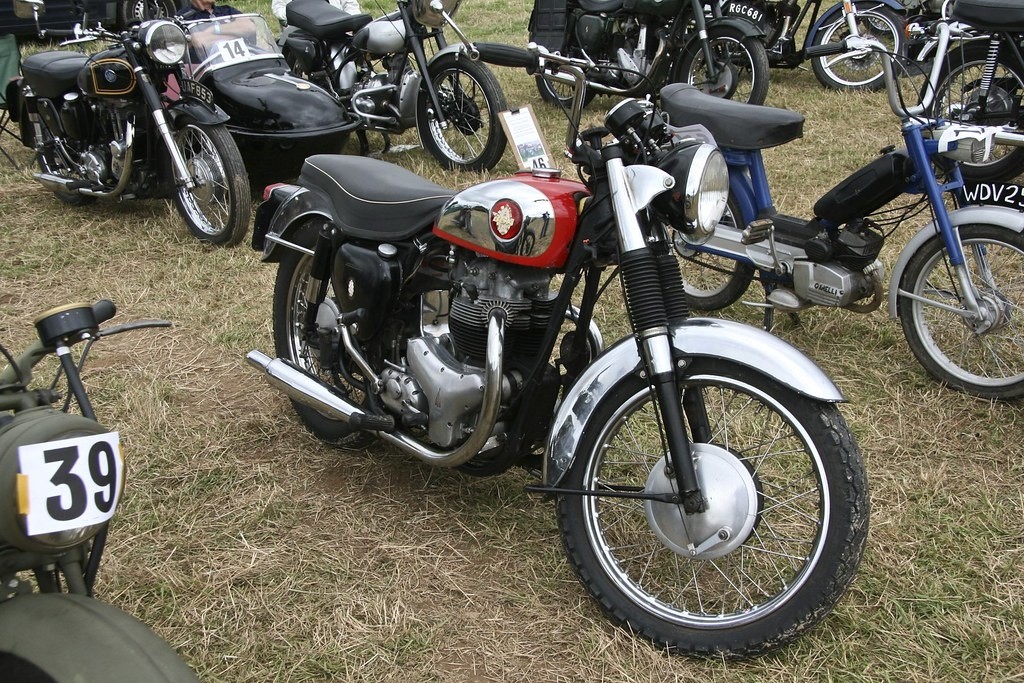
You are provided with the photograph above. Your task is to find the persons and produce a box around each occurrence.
[272,0,362,35]
[178,0,257,64]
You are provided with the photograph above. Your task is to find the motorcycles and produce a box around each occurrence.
[0,0,1023,662]
[0,296,202,683]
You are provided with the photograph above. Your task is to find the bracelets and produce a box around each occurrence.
[214,23,221,34]
